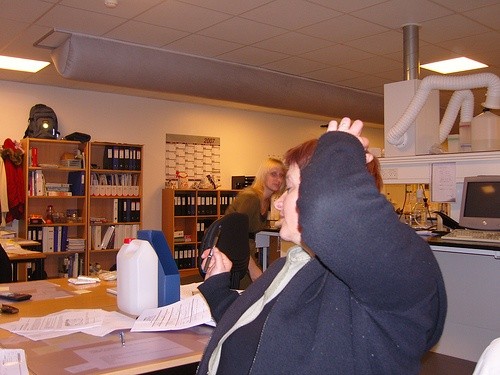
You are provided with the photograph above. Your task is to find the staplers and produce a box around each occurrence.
[0,304,19,314]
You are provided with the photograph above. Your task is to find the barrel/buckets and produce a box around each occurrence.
[116,237,159,316]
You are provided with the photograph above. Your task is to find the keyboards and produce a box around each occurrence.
[441,229,500,243]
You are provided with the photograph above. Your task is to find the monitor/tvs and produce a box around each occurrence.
[458,176,500,231]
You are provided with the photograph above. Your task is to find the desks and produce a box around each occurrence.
[0,278,216,375]
[0,239,46,281]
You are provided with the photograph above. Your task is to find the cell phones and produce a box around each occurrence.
[0,292,32,301]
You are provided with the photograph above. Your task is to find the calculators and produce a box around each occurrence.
[0,290,33,302]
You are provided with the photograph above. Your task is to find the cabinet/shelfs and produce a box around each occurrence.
[88,142,143,274]
[162,189,244,273]
[16,137,88,282]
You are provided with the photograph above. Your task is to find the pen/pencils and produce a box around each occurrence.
[120,330,125,347]
[203,225,223,272]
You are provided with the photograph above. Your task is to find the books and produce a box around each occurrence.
[174,220,217,244]
[174,246,200,270]
[89,173,138,196]
[172,193,237,216]
[25,229,86,252]
[29,147,38,167]
[26,254,84,279]
[28,170,84,197]
[104,145,141,172]
[91,225,141,250]
[111,199,139,222]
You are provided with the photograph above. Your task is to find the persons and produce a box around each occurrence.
[223,158,286,283]
[195,117,448,374]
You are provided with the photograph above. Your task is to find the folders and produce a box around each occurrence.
[173,191,239,217]
[28,226,67,252]
[103,144,142,172]
[91,225,140,250]
[174,244,200,270]
[28,169,86,196]
[197,219,217,242]
[113,198,141,223]
